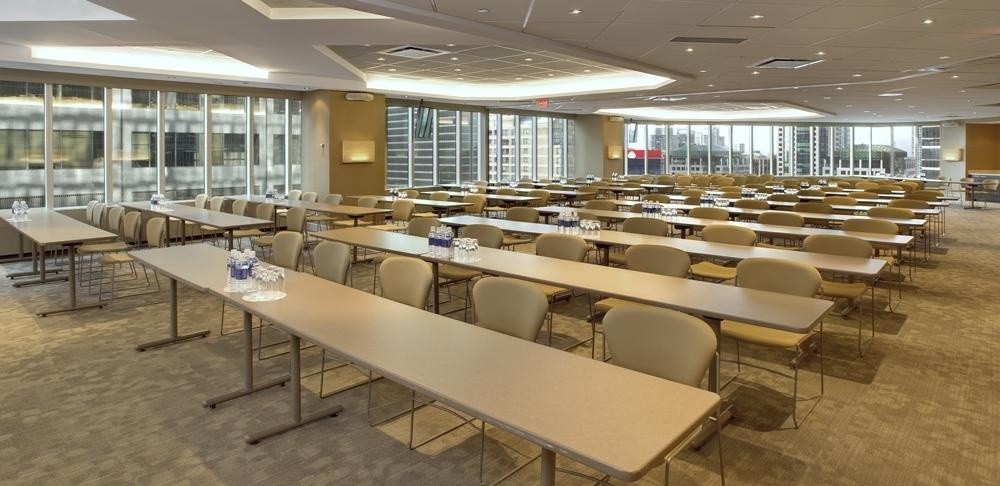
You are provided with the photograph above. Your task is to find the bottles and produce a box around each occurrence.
[800,181,809,191]
[459,182,479,196]
[151,194,164,209]
[642,200,660,218]
[428,224,455,259]
[772,184,784,196]
[741,190,756,202]
[612,172,617,180]
[700,193,713,209]
[586,174,594,183]
[389,188,398,200]
[224,249,258,293]
[556,211,580,235]
[818,180,827,189]
[265,189,278,202]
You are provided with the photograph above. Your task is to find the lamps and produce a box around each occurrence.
[342,139,375,163]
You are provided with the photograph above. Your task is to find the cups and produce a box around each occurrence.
[716,198,730,208]
[251,264,285,297]
[11,200,30,221]
[618,175,624,181]
[756,193,768,201]
[453,238,481,264]
[661,207,678,222]
[580,220,600,237]
[158,199,173,212]
[273,194,285,203]
